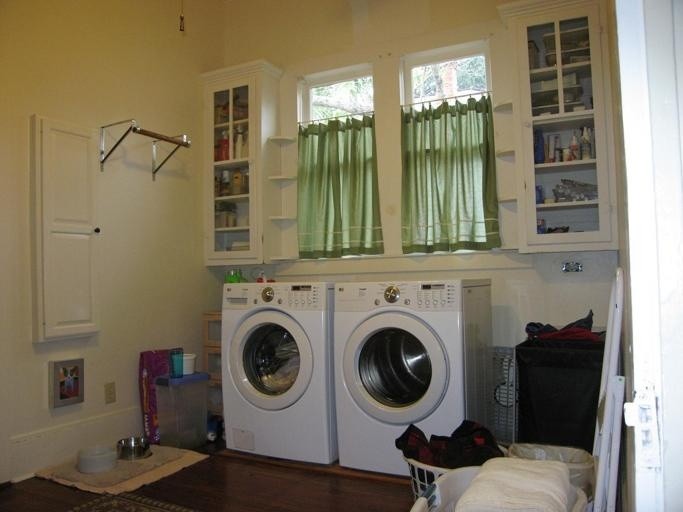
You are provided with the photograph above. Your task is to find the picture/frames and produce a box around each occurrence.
[48,358,85,408]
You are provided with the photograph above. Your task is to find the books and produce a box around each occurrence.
[231,241,250,250]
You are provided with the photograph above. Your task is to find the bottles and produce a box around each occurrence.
[215,167,250,196]
[224,269,240,284]
[534,126,594,163]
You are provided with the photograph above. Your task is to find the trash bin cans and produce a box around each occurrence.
[508,443,594,499]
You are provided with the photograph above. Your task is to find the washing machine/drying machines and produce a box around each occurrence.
[221,280,334,467]
[334,277,490,476]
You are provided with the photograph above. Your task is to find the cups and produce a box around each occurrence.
[169,349,184,378]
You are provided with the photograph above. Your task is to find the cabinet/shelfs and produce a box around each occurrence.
[486,0,620,253]
[202,312,222,416]
[196,57,298,267]
[29,111,101,343]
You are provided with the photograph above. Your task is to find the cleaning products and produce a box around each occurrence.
[569,126,595,161]
[235,125,242,158]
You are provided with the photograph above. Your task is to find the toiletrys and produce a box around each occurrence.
[537,219,545,234]
[222,130,229,160]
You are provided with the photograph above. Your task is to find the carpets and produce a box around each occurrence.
[35,443,211,497]
[66,493,197,512]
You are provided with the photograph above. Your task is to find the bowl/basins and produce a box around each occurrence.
[77,436,152,474]
[553,93,574,103]
[545,52,569,66]
